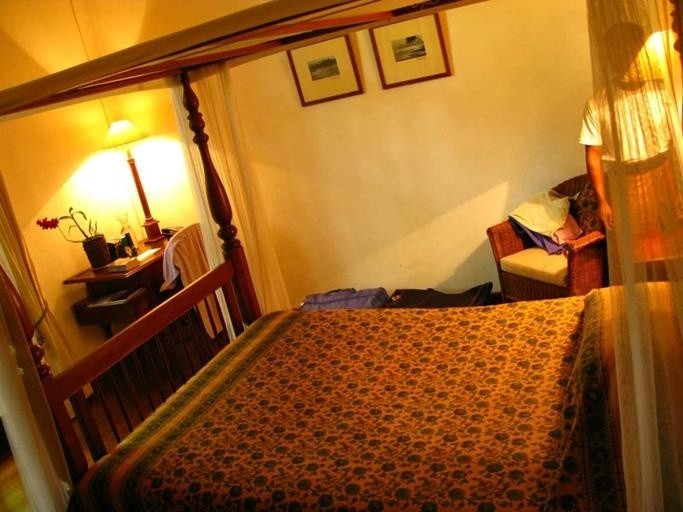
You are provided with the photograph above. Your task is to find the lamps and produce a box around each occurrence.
[97,120,165,243]
[618,29,683,85]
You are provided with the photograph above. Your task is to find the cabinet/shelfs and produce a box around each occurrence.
[62,237,210,365]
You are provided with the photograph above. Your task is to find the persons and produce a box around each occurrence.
[579,22,683,281]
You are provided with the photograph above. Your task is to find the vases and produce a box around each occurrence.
[82,233,113,269]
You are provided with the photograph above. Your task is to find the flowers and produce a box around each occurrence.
[36,205,98,244]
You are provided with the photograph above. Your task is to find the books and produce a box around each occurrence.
[105,258,139,271]
[87,287,145,308]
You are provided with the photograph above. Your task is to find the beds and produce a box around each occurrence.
[1,0,682,511]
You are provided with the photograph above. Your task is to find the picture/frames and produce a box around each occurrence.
[368,10,454,90]
[287,33,365,107]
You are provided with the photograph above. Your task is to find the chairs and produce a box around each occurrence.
[485,172,607,297]
[108,223,211,384]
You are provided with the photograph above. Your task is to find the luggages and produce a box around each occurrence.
[300,287,391,308]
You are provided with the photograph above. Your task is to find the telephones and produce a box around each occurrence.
[161,226,184,242]
